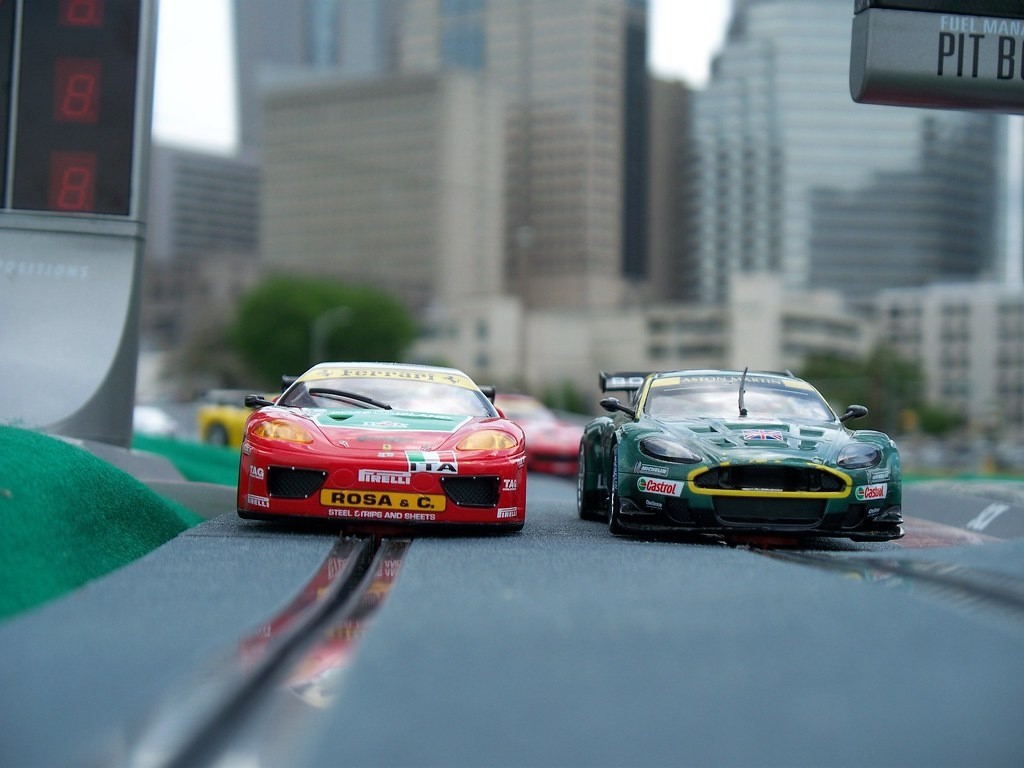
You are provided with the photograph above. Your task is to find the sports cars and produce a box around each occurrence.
[577,367,906,543]
[199,362,587,532]
